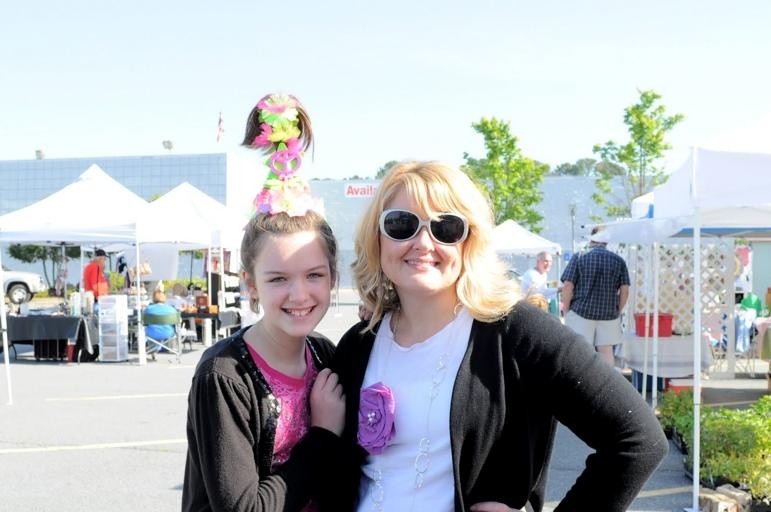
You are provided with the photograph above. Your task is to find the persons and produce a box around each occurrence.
[559,226,630,367]
[520,251,562,301]
[143,290,178,360]
[330,159,669,512]
[181,93,345,512]
[84,250,108,300]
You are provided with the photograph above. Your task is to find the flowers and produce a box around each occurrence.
[356,381,396,456]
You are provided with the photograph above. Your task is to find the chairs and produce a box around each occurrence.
[710,314,758,376]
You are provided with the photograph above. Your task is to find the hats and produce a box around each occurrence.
[94,249,110,259]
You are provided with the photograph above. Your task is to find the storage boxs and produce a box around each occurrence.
[635,313,674,337]
[668,379,705,396]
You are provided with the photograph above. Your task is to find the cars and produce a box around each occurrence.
[1,261,44,306]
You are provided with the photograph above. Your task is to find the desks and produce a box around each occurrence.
[704,317,771,360]
[627,337,711,391]
[0,302,219,362]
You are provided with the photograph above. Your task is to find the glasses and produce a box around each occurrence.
[376,205,470,247]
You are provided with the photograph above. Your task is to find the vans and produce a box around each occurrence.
[685,244,754,304]
